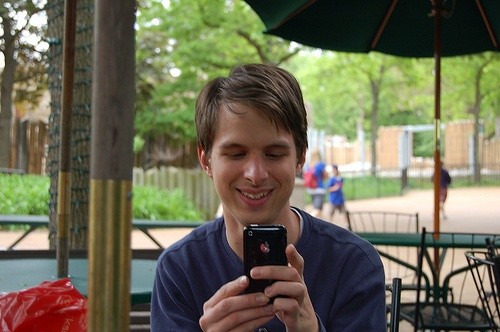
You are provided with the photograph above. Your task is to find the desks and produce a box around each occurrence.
[353,231,500,328]
[0,215,204,250]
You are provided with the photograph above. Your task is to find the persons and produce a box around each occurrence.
[431,162,451,219]
[150,63,387,332]
[302,148,347,223]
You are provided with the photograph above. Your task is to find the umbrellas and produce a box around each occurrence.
[243,0,500,303]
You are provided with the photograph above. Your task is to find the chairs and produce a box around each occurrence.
[346,211,500,332]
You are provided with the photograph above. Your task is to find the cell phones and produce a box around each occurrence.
[243,223,289,304]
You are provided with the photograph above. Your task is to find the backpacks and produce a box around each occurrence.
[302,163,319,189]
